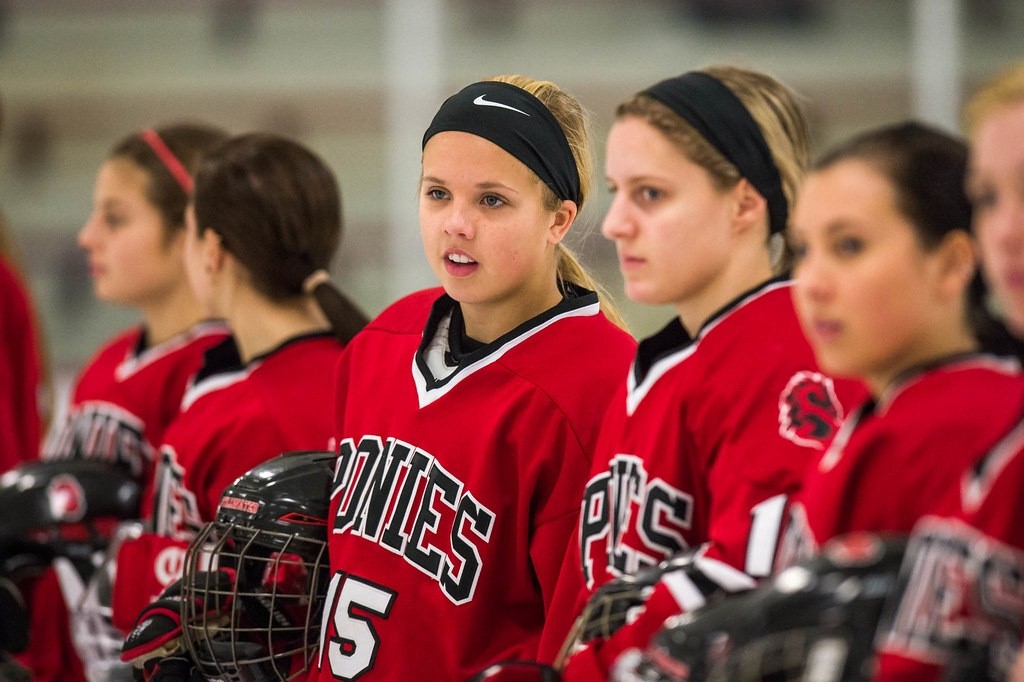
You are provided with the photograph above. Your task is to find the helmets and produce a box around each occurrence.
[627,535,904,682]
[0,457,144,560]
[554,544,705,670]
[180,451,336,682]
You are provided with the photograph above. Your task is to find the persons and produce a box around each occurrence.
[90,132,369,682]
[471,68,879,682]
[654,60,1024,682]
[0,123,236,682]
[154,74,640,682]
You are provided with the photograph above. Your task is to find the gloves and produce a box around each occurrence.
[120,568,236,669]
